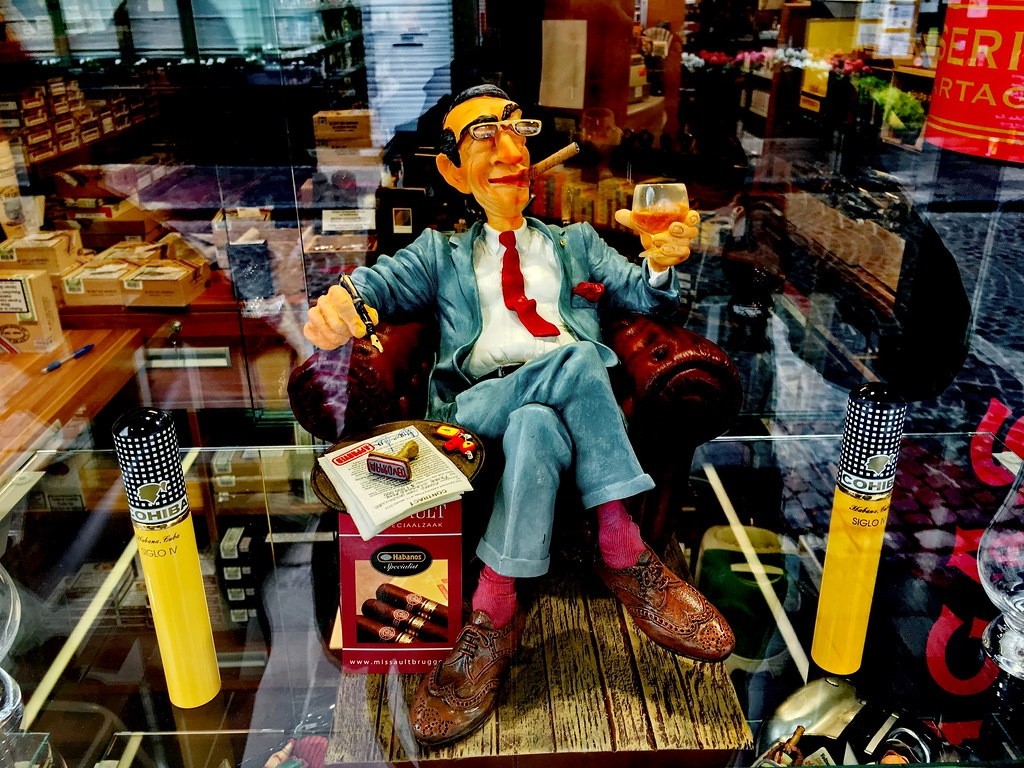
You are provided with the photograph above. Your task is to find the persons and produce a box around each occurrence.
[301,86,736,749]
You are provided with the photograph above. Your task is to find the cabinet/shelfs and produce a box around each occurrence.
[14,268,339,697]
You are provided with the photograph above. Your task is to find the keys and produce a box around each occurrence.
[435,425,476,461]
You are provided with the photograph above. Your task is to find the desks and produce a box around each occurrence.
[0,311,151,523]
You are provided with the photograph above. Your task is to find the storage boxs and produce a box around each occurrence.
[1,75,386,649]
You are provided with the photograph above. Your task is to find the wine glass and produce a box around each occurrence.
[0,563,22,722]
[631,184,689,257]
[977,457,1024,680]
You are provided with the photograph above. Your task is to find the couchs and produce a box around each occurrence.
[288,304,744,594]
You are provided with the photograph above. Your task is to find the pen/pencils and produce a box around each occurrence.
[39,342,95,376]
[338,271,385,353]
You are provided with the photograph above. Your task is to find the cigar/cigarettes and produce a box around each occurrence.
[524,142,580,178]
[351,615,425,644]
[376,583,448,628]
[361,599,448,643]
